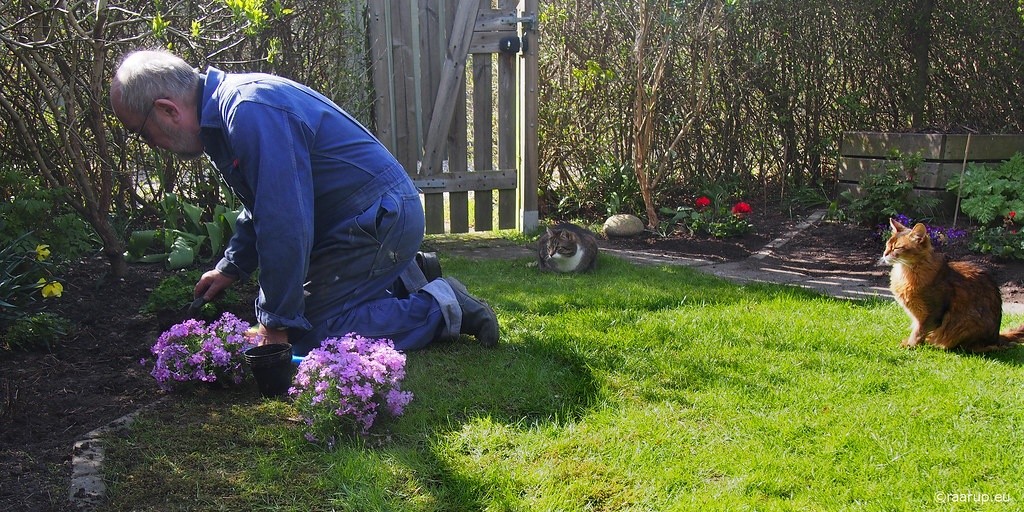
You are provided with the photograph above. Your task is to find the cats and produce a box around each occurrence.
[525,221,601,275]
[879,216,1024,353]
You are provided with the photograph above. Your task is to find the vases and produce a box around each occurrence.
[244,342,293,397]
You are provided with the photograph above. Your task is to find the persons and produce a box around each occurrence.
[109,49,500,359]
